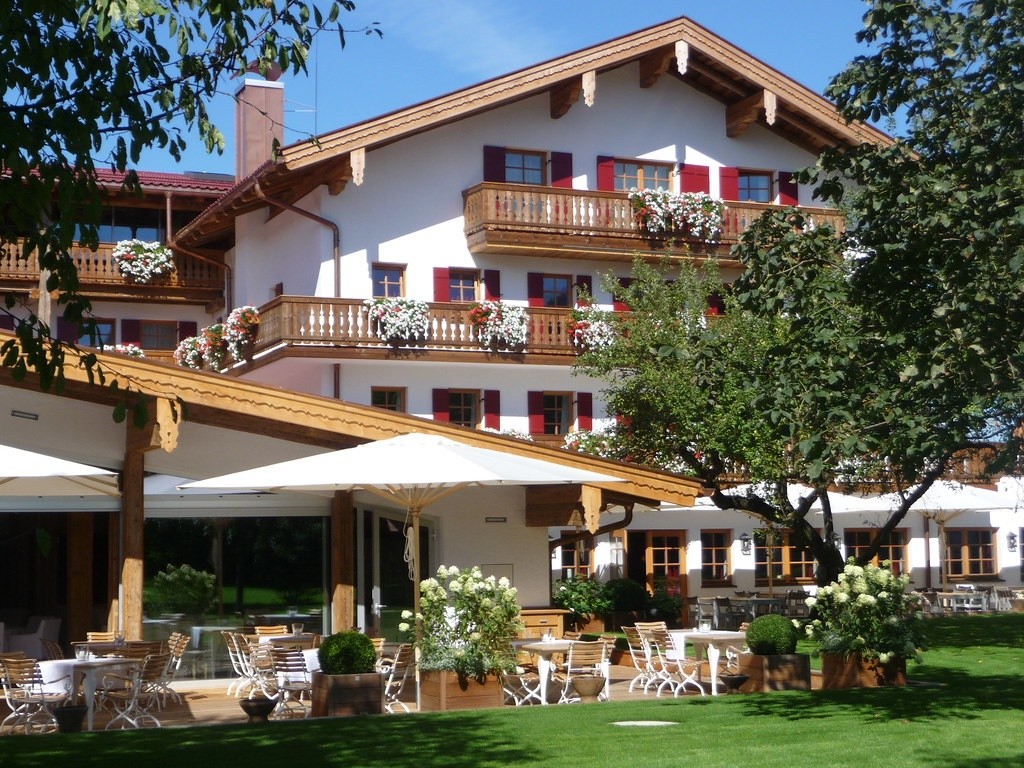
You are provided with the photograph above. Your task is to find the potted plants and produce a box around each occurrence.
[311,631,385,717]
[552,574,615,633]
[652,590,685,629]
[736,614,810,694]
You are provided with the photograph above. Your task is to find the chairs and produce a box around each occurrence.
[0,632,192,736]
[504,625,616,733]
[621,588,810,698]
[910,582,1024,612]
[220,625,414,716]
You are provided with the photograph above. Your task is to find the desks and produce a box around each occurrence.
[512,638,585,705]
[24,658,143,730]
[729,597,778,621]
[936,592,988,612]
[670,629,747,696]
[259,635,320,701]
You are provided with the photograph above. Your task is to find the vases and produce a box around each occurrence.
[420,670,504,712]
[821,653,907,689]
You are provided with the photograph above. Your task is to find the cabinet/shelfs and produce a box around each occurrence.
[521,610,572,671]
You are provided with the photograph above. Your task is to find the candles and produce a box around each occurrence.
[118,584,124,635]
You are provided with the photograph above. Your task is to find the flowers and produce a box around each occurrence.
[173,305,261,373]
[564,303,616,357]
[363,295,430,341]
[649,311,707,338]
[467,300,530,348]
[398,565,526,685]
[97,344,146,359]
[806,556,934,666]
[628,186,729,245]
[112,238,175,283]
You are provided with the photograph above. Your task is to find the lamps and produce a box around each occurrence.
[1007,531,1017,551]
[739,532,752,551]
[833,533,842,549]
